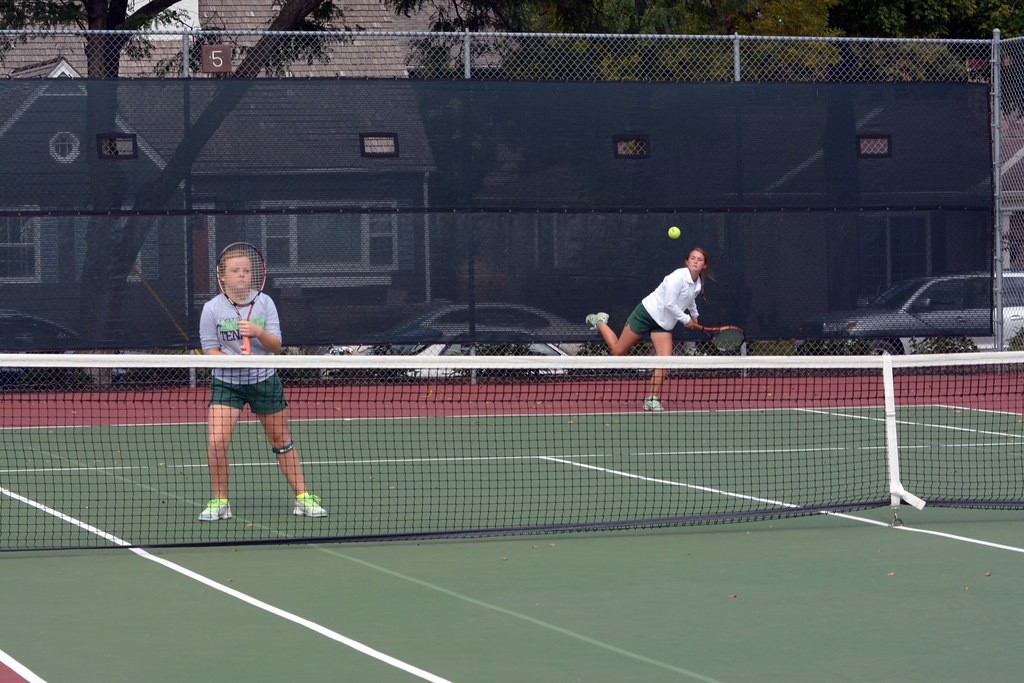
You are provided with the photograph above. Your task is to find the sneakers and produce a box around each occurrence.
[198,499,233,522]
[642,396,664,413]
[585,312,610,331]
[293,492,329,517]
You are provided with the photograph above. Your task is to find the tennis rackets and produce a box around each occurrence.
[691,322,745,352]
[216,241,267,355]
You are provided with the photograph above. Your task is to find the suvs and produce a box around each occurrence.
[822,270,1024,370]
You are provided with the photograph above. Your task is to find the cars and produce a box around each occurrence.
[317,301,703,380]
[0,311,126,386]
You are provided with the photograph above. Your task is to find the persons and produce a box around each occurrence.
[586,247,708,410]
[198,251,327,521]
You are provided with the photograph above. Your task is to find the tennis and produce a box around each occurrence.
[668,226,681,240]
